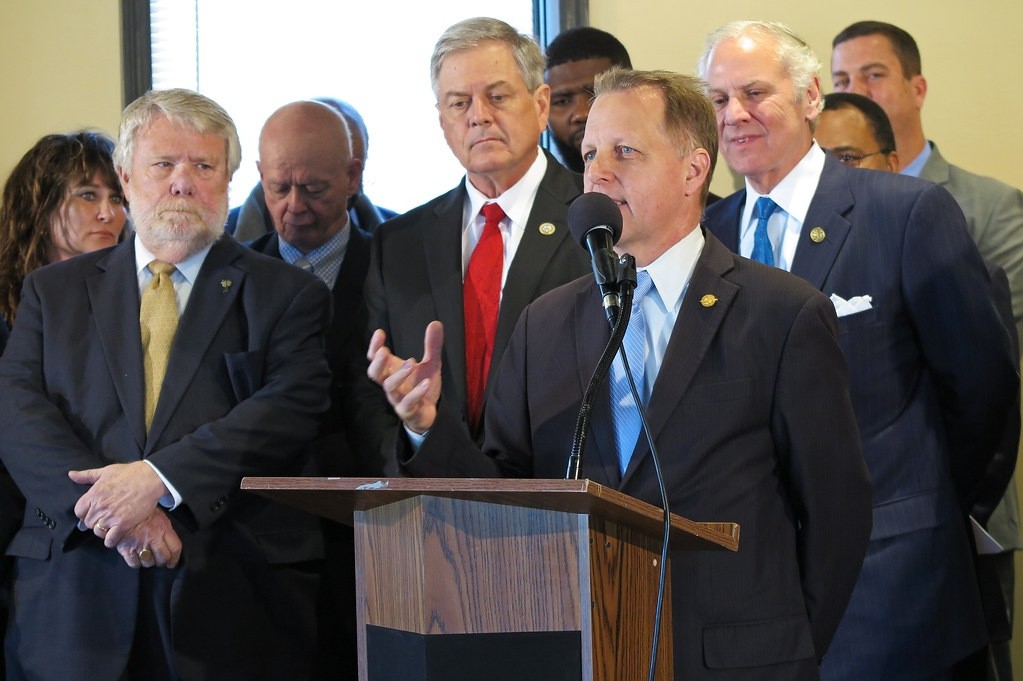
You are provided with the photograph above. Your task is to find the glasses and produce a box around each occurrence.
[835,148,888,169]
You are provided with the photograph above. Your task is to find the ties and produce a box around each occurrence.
[296,258,312,273]
[750,198,776,267]
[140,259,179,437]
[608,271,654,477]
[464,203,507,426]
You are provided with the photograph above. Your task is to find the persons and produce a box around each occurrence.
[0,18,1022,680]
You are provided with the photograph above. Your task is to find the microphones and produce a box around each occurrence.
[568,192,624,329]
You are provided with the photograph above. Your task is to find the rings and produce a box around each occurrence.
[94,520,108,534]
[136,548,153,563]
